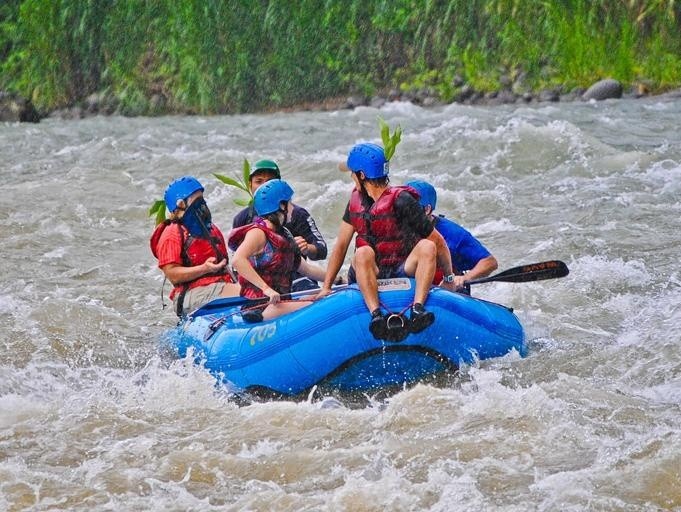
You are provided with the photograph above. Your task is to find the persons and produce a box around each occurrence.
[406,180,499,297]
[299,141,456,344]
[226,178,315,322]
[153,175,242,313]
[233,158,328,296]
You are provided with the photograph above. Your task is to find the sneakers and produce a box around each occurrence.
[369,309,386,339]
[409,303,435,333]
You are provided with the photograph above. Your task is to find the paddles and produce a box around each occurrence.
[434,260,569,290]
[181,196,237,283]
[189,284,347,318]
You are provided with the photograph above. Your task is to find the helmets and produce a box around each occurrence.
[249,159,281,178]
[251,178,294,216]
[163,175,204,212]
[339,143,389,180]
[403,180,437,210]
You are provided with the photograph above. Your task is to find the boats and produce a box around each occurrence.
[168,278,527,397]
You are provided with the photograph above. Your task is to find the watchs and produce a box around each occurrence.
[442,274,455,283]
